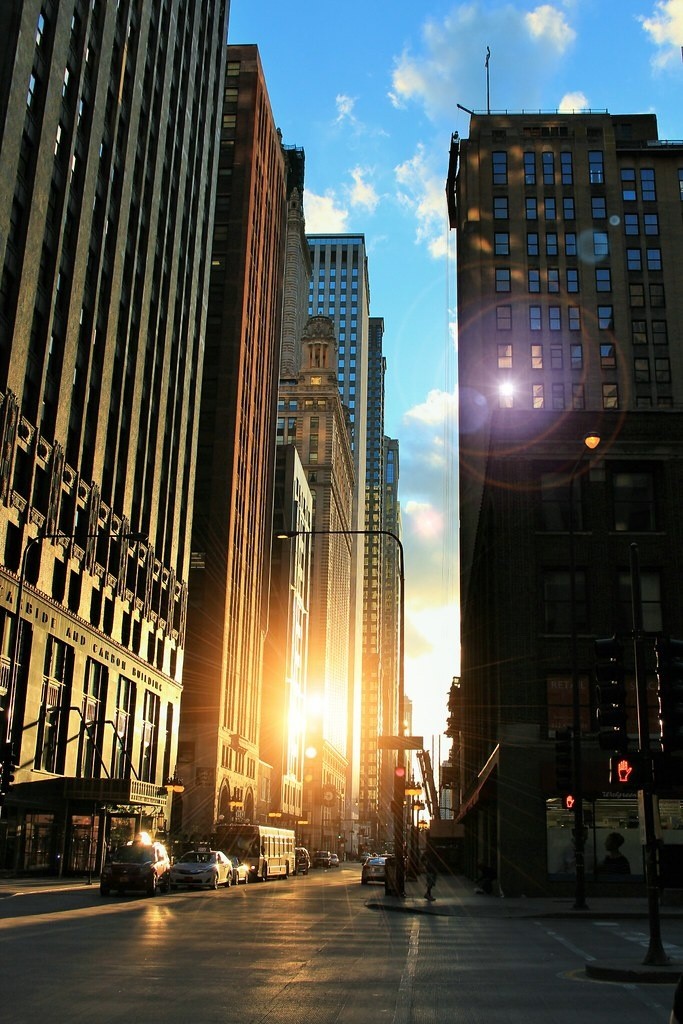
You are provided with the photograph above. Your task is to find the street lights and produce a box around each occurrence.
[349,831,354,853]
[274,523,408,899]
[0,523,149,814]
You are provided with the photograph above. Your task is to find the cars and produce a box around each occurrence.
[171,847,234,891]
[314,851,331,869]
[361,857,389,884]
[227,854,250,886]
[331,853,340,867]
[358,851,396,863]
[295,847,311,875]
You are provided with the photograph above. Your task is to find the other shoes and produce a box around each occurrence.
[425,894,436,901]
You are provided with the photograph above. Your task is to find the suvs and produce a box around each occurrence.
[99,843,173,899]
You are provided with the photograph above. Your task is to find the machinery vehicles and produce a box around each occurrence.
[411,745,500,872]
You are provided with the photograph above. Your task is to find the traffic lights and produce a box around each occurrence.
[393,765,405,806]
[555,730,576,810]
[608,757,647,792]
[590,634,628,754]
[337,835,342,843]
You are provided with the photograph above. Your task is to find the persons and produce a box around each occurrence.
[420,840,440,902]
[552,824,631,876]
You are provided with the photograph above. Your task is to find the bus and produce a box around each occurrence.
[216,824,296,882]
